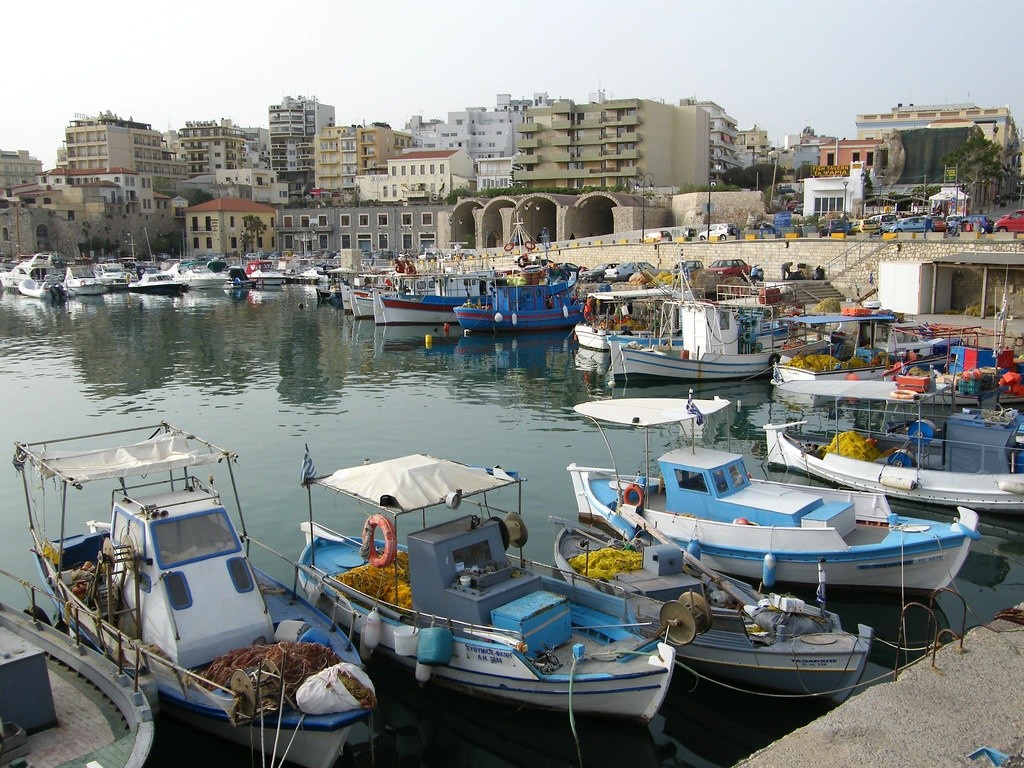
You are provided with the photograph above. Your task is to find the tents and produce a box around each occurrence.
[929,187,973,217]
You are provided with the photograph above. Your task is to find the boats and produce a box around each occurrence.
[341,207,1023,410]
[373,212,581,325]
[762,375,1024,517]
[0,251,360,299]
[566,389,979,598]
[13,420,380,768]
[0,570,155,768]
[295,442,714,722]
[548,507,875,707]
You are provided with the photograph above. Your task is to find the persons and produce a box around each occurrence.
[780,262,793,280]
[895,204,948,219]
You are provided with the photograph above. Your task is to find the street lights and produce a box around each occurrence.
[634,173,655,242]
[400,219,412,261]
[955,178,992,217]
[1019,180,1024,209]
[448,212,463,242]
[524,196,541,252]
[707,147,723,237]
[843,182,849,219]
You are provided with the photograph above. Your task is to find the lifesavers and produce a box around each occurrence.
[891,390,918,399]
[406,265,415,274]
[788,311,803,331]
[396,265,401,272]
[81,281,85,286]
[614,311,629,323]
[624,484,644,507]
[139,268,145,275]
[584,307,602,323]
[768,353,781,370]
[361,513,397,569]
[518,256,527,267]
[525,241,536,250]
[503,242,514,251]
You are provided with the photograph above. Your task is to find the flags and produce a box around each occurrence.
[686,392,704,426]
[301,450,317,487]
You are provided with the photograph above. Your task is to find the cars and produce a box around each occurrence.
[952,216,989,234]
[643,200,1024,237]
[155,246,443,263]
[945,215,962,227]
[994,210,1024,233]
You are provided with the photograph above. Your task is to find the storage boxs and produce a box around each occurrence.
[491,591,573,660]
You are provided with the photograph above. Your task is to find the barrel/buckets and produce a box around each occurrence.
[681,349,689,358]
[759,287,781,303]
[274,620,330,647]
[393,626,419,656]
[460,575,471,588]
[418,628,454,666]
[997,372,1024,397]
